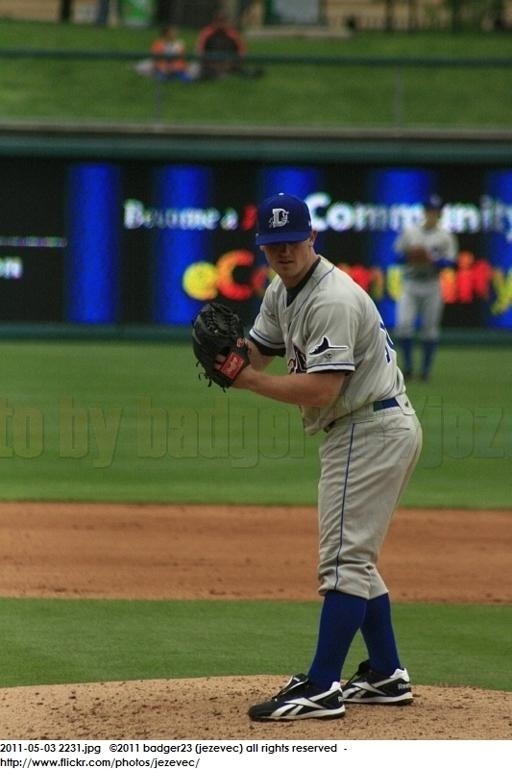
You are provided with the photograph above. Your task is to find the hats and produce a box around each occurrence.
[253,192,312,246]
[424,192,444,211]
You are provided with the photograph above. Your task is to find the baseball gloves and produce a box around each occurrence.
[192,303,251,388]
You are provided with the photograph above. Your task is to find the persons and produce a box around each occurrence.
[392,193,461,383]
[190,194,424,723]
[145,5,264,84]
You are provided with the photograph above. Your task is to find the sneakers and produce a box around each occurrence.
[341,659,415,707]
[247,673,346,723]
